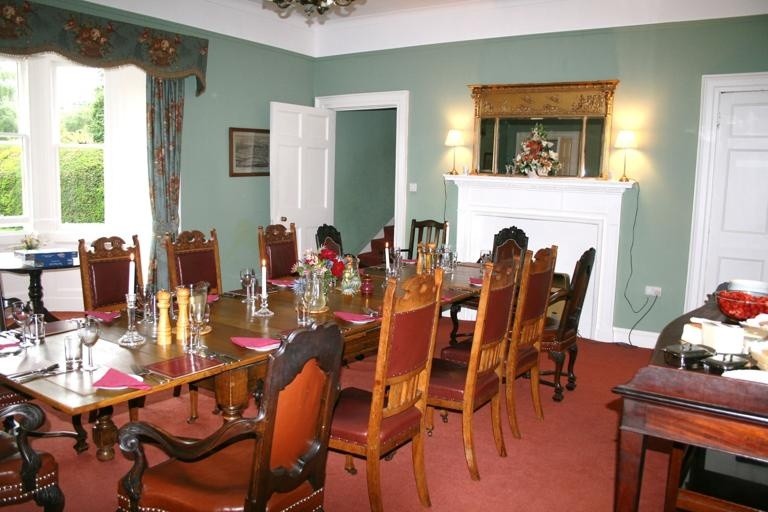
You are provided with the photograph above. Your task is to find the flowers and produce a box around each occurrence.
[509,126,561,177]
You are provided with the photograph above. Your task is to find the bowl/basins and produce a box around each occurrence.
[713,288,767,322]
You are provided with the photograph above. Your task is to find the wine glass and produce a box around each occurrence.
[11,301,36,350]
[135,283,155,327]
[387,245,401,279]
[240,268,256,306]
[189,284,209,332]
[76,318,104,372]
[157,255,184,322]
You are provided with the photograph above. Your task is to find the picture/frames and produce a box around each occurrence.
[227,128,271,178]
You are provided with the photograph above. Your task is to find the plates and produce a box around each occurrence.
[349,314,375,325]
[466,278,483,286]
[244,342,281,354]
[85,311,123,321]
[268,280,293,290]
[97,372,144,389]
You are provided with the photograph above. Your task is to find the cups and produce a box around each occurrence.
[63,336,83,372]
[439,247,456,276]
[27,314,45,345]
[183,321,200,355]
[295,292,312,326]
[144,300,161,339]
[479,250,492,276]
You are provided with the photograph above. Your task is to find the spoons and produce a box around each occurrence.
[9,364,62,378]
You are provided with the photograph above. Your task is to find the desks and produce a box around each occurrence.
[606,276,768,512]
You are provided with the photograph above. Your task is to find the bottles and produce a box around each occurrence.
[413,242,438,274]
[118,292,146,349]
[361,274,373,300]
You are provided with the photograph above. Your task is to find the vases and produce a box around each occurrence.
[527,168,542,178]
